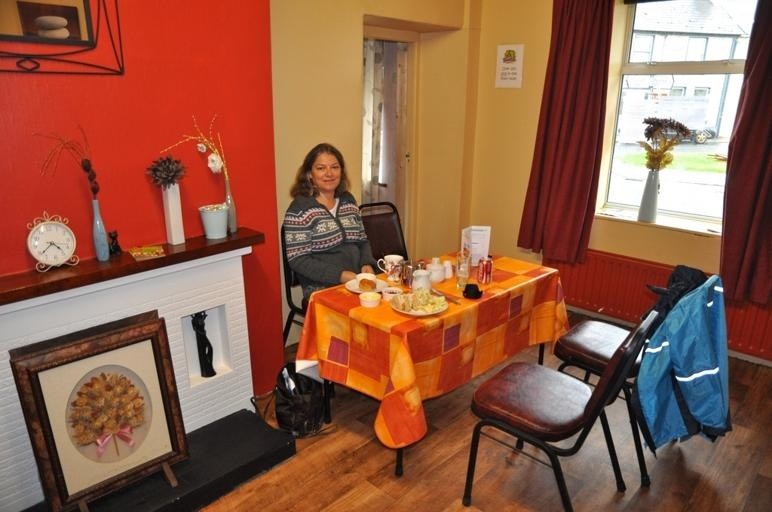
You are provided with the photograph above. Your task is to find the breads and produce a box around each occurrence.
[359,279,376,291]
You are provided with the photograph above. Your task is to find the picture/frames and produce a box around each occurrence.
[7,314,191,512]
[0,0,129,80]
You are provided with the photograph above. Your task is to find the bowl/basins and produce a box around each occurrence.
[356,273,377,287]
[358,292,382,308]
[382,288,403,301]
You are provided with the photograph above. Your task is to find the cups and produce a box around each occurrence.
[456,253,471,289]
[199,205,229,240]
[377,254,404,282]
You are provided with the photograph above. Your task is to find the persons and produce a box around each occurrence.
[283,142,378,303]
[192,311,217,377]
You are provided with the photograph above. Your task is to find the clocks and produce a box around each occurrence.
[23,208,79,275]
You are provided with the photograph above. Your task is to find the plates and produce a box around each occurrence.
[391,295,449,316]
[344,280,388,293]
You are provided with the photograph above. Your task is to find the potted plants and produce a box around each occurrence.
[147,152,189,246]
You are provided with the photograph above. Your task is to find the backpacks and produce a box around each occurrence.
[272,364,327,437]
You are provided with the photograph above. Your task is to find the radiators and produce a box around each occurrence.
[538,245,772,363]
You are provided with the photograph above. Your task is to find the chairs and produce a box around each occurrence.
[462,307,664,512]
[550,264,722,495]
[281,219,337,402]
[355,199,414,273]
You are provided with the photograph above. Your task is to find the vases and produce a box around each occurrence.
[223,177,238,235]
[638,166,661,224]
[92,196,112,262]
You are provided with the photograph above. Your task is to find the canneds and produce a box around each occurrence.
[405,266,416,287]
[477,257,492,285]
[416,262,424,270]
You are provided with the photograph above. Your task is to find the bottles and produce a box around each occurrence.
[402,256,453,292]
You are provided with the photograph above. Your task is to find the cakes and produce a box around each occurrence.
[392,287,430,310]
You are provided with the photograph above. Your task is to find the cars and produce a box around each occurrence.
[652,116,718,145]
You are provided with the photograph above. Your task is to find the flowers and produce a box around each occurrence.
[636,115,694,172]
[159,114,227,175]
[28,121,103,198]
